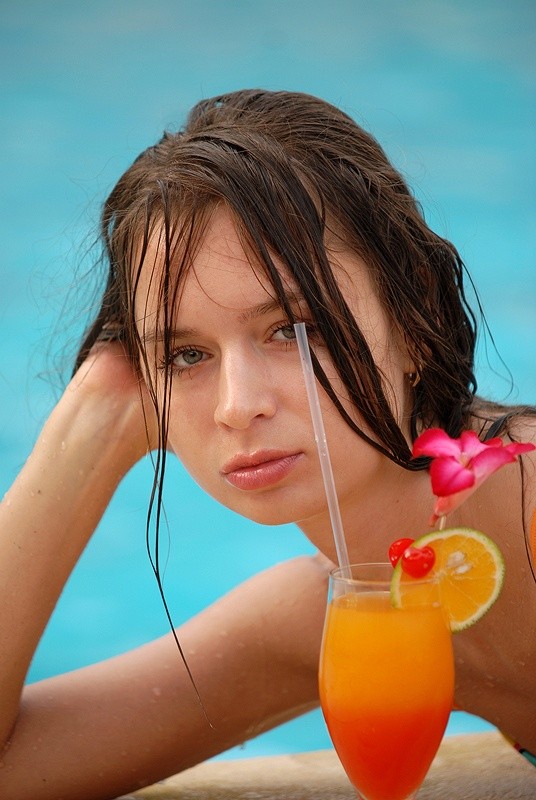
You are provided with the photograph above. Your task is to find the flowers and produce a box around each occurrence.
[410,424,536,525]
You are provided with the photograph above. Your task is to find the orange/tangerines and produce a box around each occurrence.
[390,527,506,632]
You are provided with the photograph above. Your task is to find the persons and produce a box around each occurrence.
[0,88,536,800]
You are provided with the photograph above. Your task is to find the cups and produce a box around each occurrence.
[318,562,454,800]
